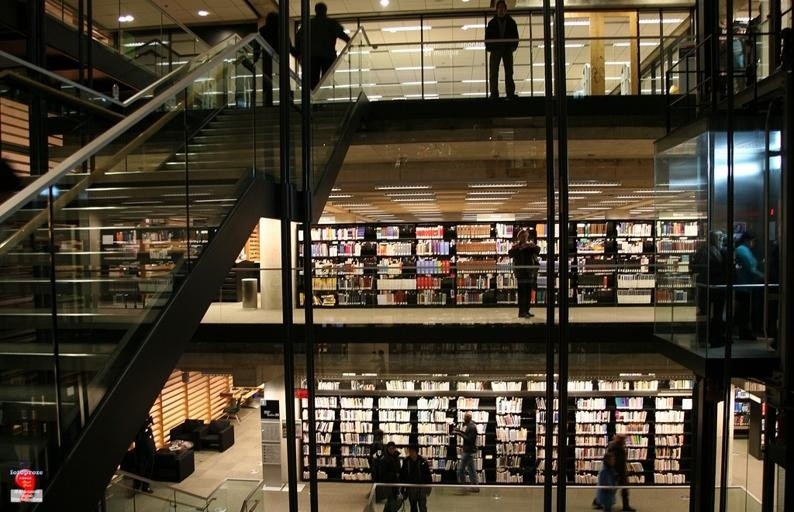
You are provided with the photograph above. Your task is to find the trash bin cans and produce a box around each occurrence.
[241,278,257,309]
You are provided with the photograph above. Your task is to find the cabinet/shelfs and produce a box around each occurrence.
[734,384,779,460]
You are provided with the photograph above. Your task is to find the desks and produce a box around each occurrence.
[155,446,194,483]
[156,440,194,451]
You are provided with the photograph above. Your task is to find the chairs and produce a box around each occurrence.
[170,419,234,453]
[223,395,242,424]
[218,263,260,302]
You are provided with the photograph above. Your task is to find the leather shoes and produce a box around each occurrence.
[519,311,535,318]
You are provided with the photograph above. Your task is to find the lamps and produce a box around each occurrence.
[101,225,217,304]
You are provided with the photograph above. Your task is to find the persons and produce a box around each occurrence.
[252,12,295,107]
[451,414,480,495]
[379,441,401,511]
[297,4,351,103]
[703,230,765,348]
[483,0,520,99]
[733,224,745,240]
[126,412,157,499]
[397,445,433,512]
[594,453,618,512]
[605,433,637,512]
[508,231,541,318]
[368,430,384,503]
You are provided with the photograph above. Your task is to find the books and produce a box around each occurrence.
[300,379,694,485]
[298,222,701,306]
